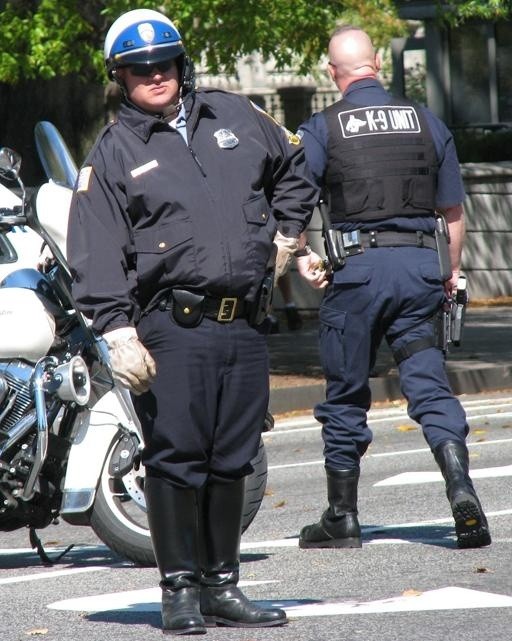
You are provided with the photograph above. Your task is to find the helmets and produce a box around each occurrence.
[103,9,186,83]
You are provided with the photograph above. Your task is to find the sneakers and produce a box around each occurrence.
[286,305,303,329]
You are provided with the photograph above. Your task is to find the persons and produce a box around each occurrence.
[294,26,492,550]
[66,8,321,635]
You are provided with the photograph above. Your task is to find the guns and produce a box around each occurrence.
[451,277,469,346]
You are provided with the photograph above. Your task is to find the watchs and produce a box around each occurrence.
[294,241,312,257]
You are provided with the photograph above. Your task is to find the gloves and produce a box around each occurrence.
[100,326,157,396]
[271,230,299,288]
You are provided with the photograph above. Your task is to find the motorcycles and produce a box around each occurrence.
[1,120,274,568]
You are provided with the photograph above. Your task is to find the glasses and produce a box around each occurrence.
[128,60,171,77]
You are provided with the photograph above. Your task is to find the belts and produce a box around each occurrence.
[360,232,437,249]
[196,297,245,322]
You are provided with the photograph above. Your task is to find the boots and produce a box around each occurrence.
[298,469,363,548]
[200,478,286,628]
[144,477,207,633]
[433,440,492,548]
